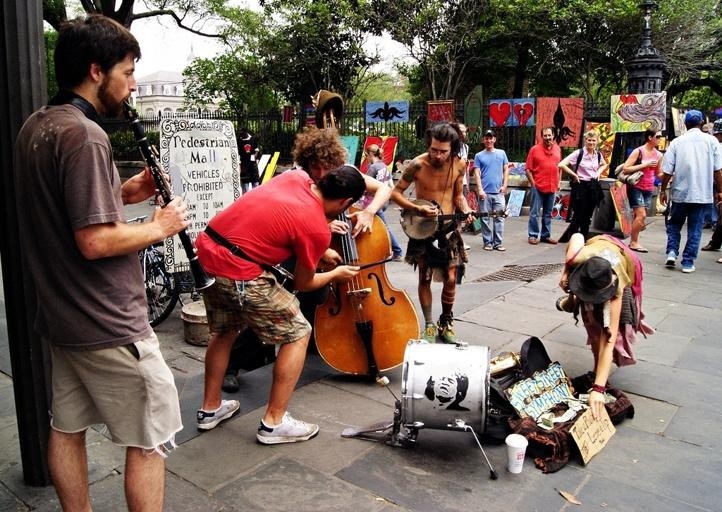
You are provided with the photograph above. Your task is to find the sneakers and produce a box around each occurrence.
[575,371,596,382]
[421,322,457,343]
[701,243,720,249]
[527,235,538,244]
[254,410,320,443]
[195,399,240,430]
[540,235,557,244]
[682,261,696,274]
[664,249,678,268]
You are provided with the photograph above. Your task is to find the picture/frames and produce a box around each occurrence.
[424,98,457,133]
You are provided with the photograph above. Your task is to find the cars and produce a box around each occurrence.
[354,126,385,135]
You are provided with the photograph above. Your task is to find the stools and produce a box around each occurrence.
[177,302,212,348]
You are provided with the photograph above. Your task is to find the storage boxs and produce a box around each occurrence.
[234,327,276,373]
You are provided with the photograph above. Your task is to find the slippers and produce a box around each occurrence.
[482,243,506,250]
[628,242,650,254]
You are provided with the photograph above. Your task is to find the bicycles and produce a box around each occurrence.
[126,215,204,328]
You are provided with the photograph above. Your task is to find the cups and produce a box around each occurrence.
[505,433,530,473]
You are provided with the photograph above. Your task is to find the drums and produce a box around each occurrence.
[401,339,491,435]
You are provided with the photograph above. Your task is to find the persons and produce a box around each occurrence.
[424,365,471,411]
[364,144,404,261]
[624,110,721,272]
[12,13,191,512]
[526,127,608,245]
[458,124,509,252]
[237,131,261,192]
[296,126,392,239]
[191,166,368,445]
[392,120,478,346]
[557,230,654,421]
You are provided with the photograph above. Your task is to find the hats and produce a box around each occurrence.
[684,109,703,124]
[567,255,620,305]
[483,129,497,139]
[708,118,721,134]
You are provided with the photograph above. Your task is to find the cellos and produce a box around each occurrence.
[314,105,420,377]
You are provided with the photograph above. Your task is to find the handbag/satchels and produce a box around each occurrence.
[614,148,644,185]
